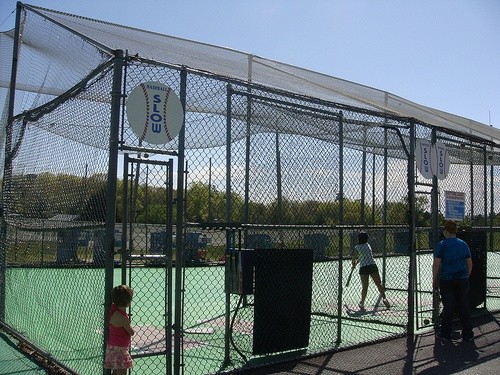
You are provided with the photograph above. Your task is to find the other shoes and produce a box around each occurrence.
[461,333,473,341]
[359,303,364,309]
[436,334,450,342]
[383,298,391,309]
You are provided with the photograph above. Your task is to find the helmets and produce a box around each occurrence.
[358,232,370,240]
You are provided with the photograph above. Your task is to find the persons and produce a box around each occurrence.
[351,231,391,314]
[104,284,135,375]
[431,219,472,341]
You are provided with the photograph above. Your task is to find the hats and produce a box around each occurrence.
[444,219,457,233]
